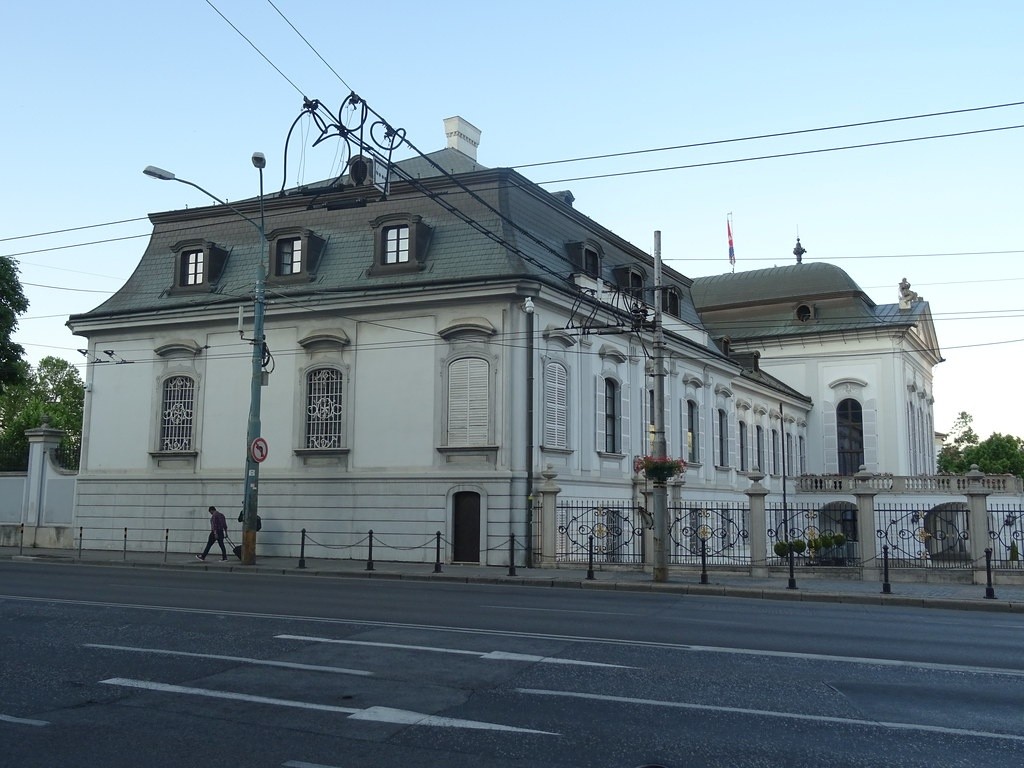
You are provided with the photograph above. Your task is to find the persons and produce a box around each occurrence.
[238,500,244,523]
[196,506,229,563]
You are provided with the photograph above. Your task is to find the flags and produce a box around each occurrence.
[726,212,736,264]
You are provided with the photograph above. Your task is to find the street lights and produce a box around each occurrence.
[141,151,267,568]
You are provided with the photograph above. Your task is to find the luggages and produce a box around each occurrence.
[225,537,241,560]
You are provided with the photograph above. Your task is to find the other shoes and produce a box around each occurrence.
[196,555,204,562]
[219,559,228,562]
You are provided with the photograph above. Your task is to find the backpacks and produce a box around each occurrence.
[256,514,262,532]
